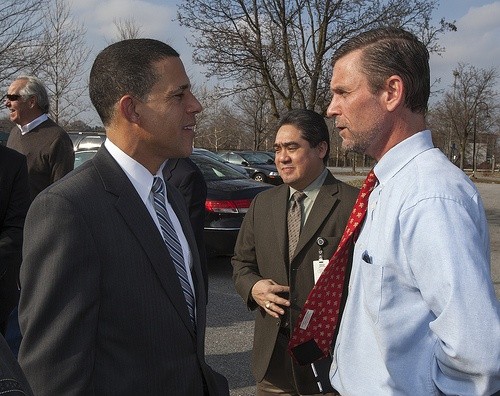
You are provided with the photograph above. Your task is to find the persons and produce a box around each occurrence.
[0,144,30,359]
[4,73,75,203]
[17,38,231,396]
[287,27,500,396]
[230,109,362,396]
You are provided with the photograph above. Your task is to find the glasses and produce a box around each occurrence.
[6,93,23,101]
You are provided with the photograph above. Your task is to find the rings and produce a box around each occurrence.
[265,303,271,308]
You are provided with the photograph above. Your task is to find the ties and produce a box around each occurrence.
[151,176,198,332]
[284,168,377,368]
[288,190,308,264]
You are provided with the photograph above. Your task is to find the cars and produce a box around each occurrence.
[67,131,282,249]
